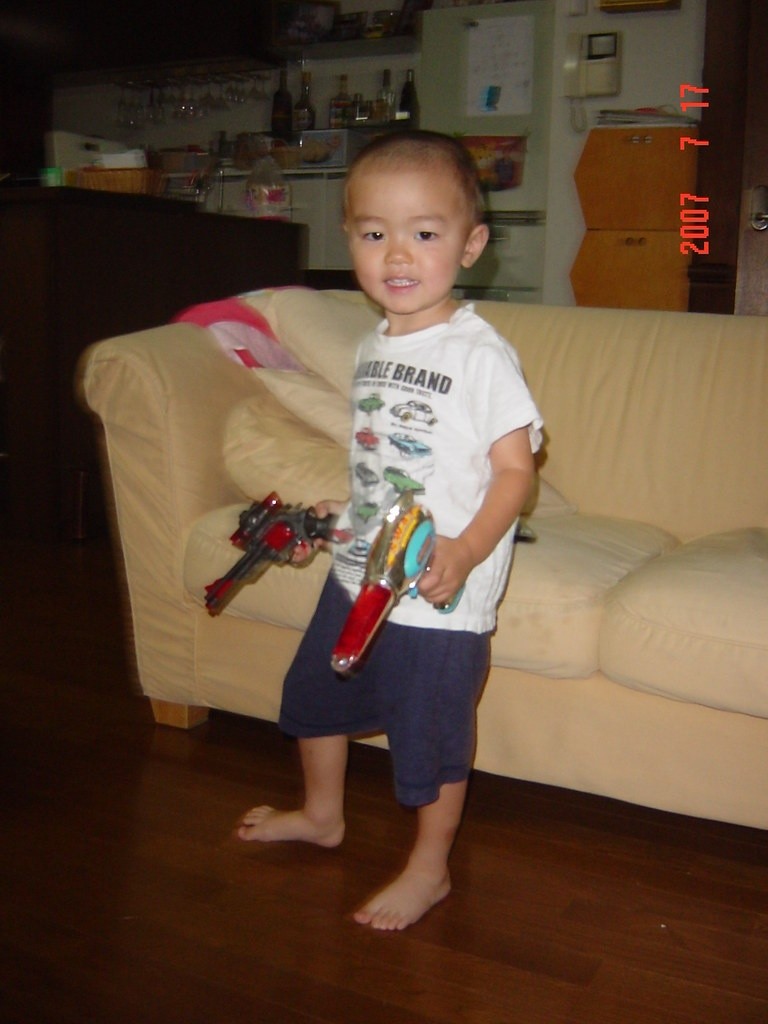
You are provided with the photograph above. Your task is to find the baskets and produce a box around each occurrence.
[78,167,164,197]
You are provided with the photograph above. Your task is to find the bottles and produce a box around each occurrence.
[397,69,420,127]
[270,65,292,130]
[328,74,351,129]
[373,68,395,125]
[293,71,315,131]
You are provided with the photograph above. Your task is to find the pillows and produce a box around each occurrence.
[224,288,579,516]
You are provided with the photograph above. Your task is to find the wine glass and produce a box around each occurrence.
[114,76,271,129]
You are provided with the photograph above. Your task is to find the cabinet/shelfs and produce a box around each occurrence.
[47,34,421,270]
[0,184,309,539]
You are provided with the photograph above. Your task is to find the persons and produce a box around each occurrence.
[239,130,545,930]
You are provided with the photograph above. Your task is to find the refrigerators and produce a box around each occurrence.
[413,5,552,306]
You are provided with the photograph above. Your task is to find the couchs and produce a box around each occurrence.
[83,280,768,830]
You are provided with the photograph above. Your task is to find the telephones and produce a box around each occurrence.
[561,30,624,97]
